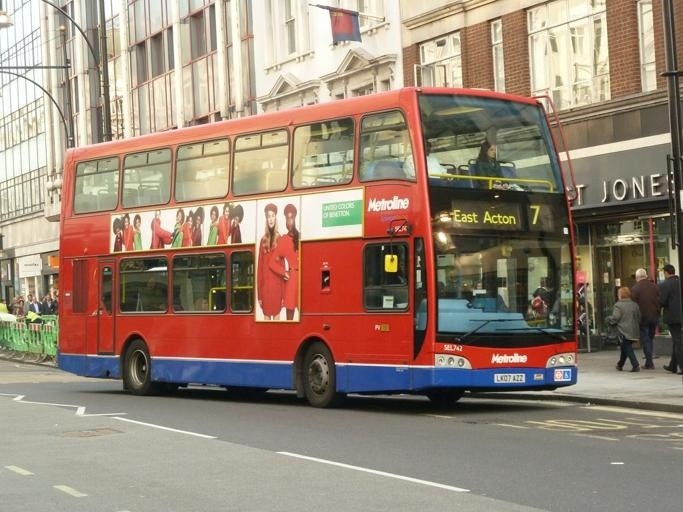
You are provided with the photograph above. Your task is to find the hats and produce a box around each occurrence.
[284,204,297,216]
[265,204,277,213]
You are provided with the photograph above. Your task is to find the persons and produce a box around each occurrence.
[136,276,165,310]
[401,140,453,181]
[528,277,558,319]
[601,265,683,373]
[477,141,509,190]
[0,291,59,343]
[194,296,208,311]
[473,280,483,289]
[256,202,299,320]
[112,202,244,252]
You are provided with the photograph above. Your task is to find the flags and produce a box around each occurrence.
[317,4,362,47]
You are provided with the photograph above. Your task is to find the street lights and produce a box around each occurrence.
[0,1,117,198]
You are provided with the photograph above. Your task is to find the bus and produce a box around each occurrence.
[54,88,579,405]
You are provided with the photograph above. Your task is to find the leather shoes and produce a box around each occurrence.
[615,362,683,375]
